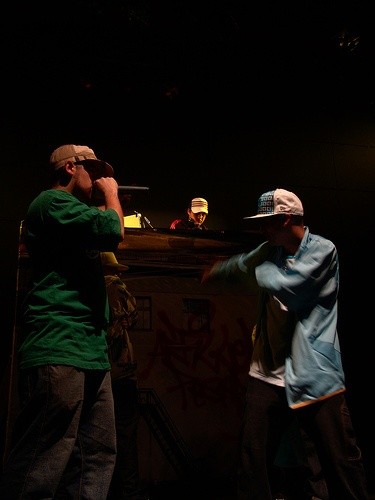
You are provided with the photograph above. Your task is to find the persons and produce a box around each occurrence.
[0,142,126,500]
[168,197,209,232]
[100,250,151,500]
[199,186,368,500]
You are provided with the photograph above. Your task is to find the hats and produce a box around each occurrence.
[189,197,209,214]
[49,144,113,180]
[243,188,304,219]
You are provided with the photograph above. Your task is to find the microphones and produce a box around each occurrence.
[118,186,149,194]
